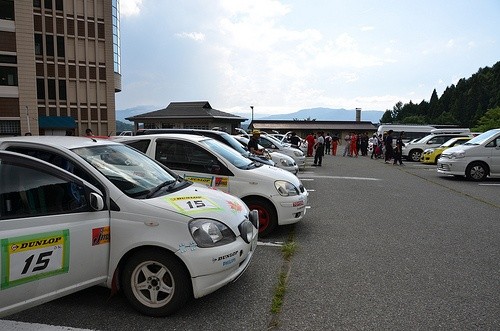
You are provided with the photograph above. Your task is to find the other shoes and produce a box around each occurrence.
[311,163,318,167]
[385,161,392,164]
[317,163,321,166]
[393,163,399,165]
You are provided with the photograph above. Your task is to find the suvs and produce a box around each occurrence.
[402,134,470,162]
[135,129,276,181]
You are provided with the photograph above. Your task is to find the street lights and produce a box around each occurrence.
[250,105,255,135]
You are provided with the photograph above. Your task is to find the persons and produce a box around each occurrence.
[287,132,302,148]
[248,130,263,155]
[303,130,407,167]
[85,129,94,136]
[25,133,32,136]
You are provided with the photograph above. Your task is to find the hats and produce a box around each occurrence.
[252,129,262,135]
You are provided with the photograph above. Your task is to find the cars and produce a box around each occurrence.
[110,136,309,237]
[0,136,259,314]
[235,129,308,174]
[365,137,374,154]
[119,131,133,137]
[436,129,500,181]
[421,137,472,166]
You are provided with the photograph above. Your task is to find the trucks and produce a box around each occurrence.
[431,129,471,136]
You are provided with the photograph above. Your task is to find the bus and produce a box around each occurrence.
[378,124,436,154]
